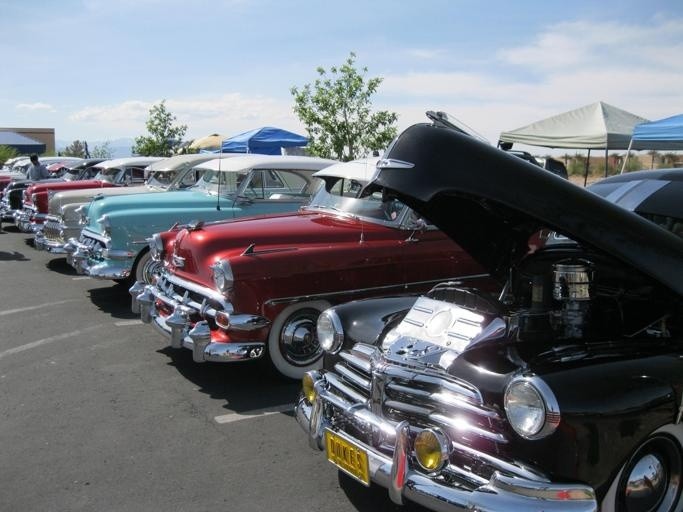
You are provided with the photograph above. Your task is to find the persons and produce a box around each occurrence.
[25,152,51,182]
